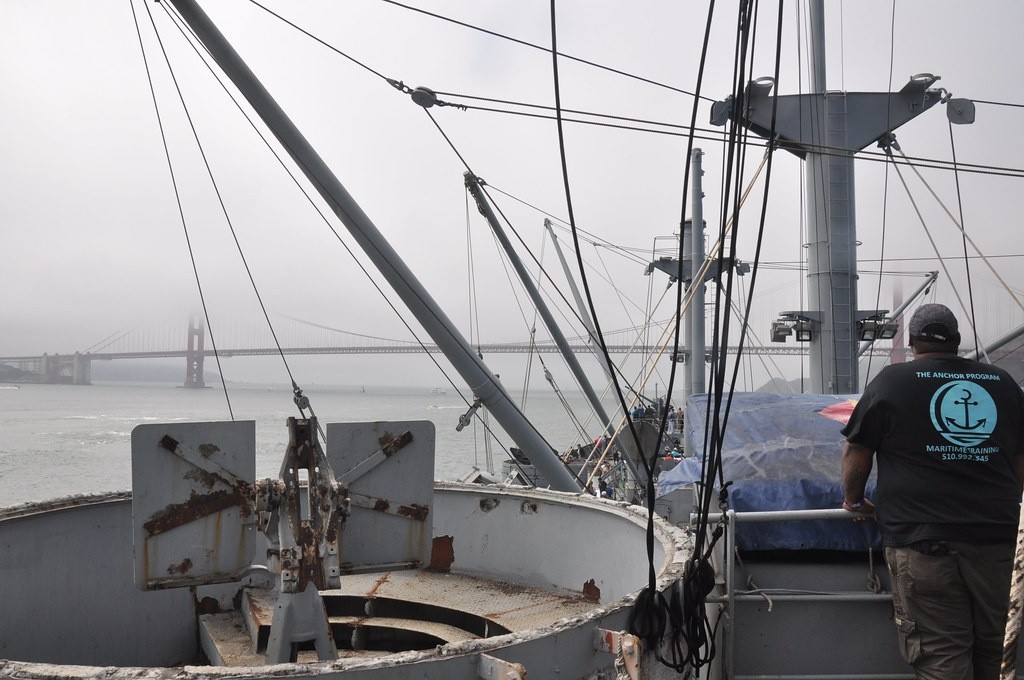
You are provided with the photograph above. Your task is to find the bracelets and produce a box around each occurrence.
[844,498,865,509]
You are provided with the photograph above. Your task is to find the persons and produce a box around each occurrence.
[629,403,684,434]
[839,303,1024,680]
[659,445,683,460]
[571,435,625,500]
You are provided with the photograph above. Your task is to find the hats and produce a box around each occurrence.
[908,304,958,346]
